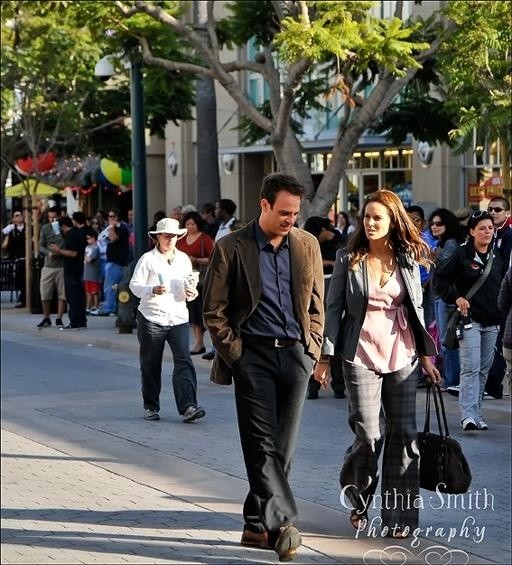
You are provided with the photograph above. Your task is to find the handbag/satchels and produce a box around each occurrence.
[197,239,208,289]
[422,320,439,376]
[418,379,472,494]
[441,307,461,350]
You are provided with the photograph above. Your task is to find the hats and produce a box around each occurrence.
[148,217,189,242]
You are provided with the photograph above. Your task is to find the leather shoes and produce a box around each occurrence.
[271,524,302,562]
[306,390,319,400]
[190,347,206,355]
[334,391,346,399]
[240,525,271,549]
[202,352,215,360]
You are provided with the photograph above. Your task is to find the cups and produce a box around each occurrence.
[183,273,196,303]
[461,310,473,332]
[51,221,61,234]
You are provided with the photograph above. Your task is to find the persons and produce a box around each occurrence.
[2,170,512,562]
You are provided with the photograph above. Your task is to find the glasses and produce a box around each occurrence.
[486,207,505,213]
[429,221,444,227]
[472,211,489,219]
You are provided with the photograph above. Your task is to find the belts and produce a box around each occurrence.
[267,338,295,351]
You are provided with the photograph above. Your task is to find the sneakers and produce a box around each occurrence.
[462,416,478,431]
[182,406,206,422]
[479,421,489,429]
[90,309,100,316]
[143,408,160,420]
[446,384,460,395]
[36,318,87,331]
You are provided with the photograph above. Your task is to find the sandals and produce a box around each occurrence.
[349,508,369,532]
[389,529,408,538]
